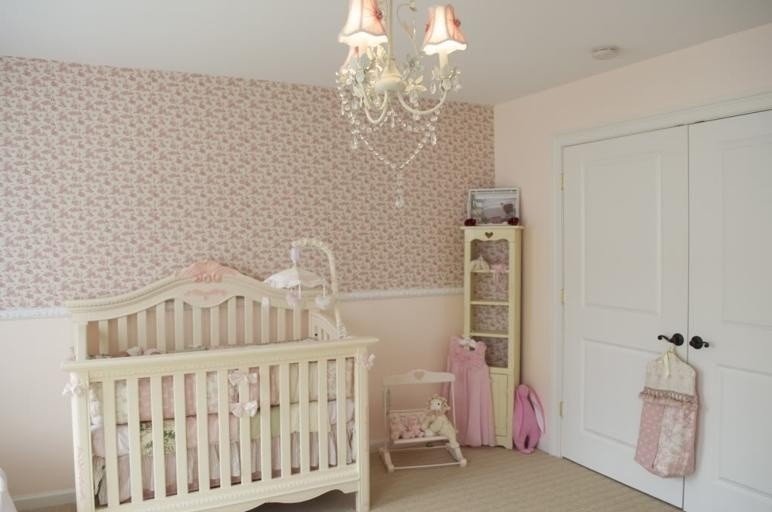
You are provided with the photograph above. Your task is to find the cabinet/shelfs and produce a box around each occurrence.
[458,223,524,452]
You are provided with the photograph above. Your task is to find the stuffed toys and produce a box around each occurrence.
[421,395,459,451]
[407,414,424,438]
[391,416,410,441]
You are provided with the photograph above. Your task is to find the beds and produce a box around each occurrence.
[58,258,381,511]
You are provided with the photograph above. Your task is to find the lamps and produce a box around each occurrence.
[330,0,470,208]
